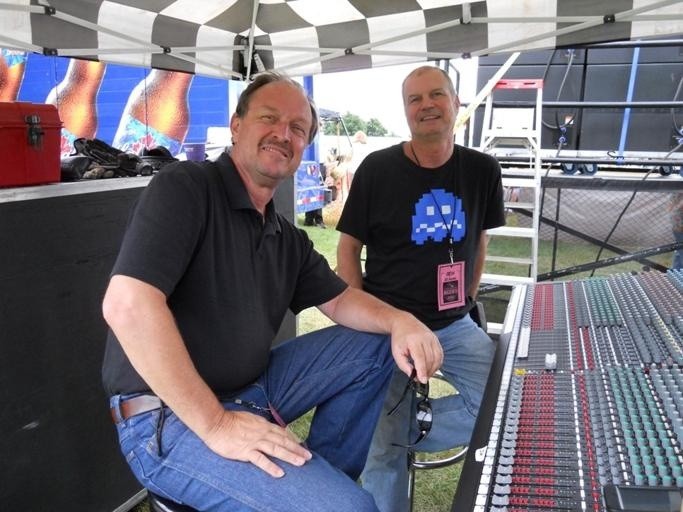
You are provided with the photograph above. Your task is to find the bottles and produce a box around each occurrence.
[46,60,189,162]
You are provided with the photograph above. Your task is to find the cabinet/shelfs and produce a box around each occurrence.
[0,175,154,512]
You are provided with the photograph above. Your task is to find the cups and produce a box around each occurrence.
[182,141,207,163]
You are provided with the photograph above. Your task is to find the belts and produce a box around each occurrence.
[111,394,166,426]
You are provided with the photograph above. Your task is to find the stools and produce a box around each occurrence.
[334,272,487,471]
[139,487,201,512]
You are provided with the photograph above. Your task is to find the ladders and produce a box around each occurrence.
[479,79,543,335]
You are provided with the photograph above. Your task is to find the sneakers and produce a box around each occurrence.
[304,222,326,228]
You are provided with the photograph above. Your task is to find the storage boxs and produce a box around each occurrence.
[0,99,64,188]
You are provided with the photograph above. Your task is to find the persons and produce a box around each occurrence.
[98,69,444,512]
[335,65,507,511]
[305,119,372,231]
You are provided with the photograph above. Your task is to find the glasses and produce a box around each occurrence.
[387,369,433,448]
